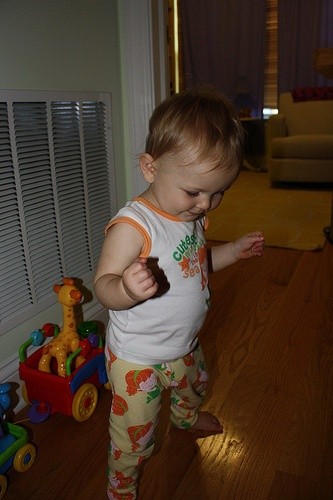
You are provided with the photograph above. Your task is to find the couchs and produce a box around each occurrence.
[268,94,333,189]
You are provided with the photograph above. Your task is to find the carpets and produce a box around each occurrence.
[202,170,333,251]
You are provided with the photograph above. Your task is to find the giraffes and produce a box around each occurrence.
[37,277,86,378]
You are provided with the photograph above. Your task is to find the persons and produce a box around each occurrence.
[96,90,266,500]
